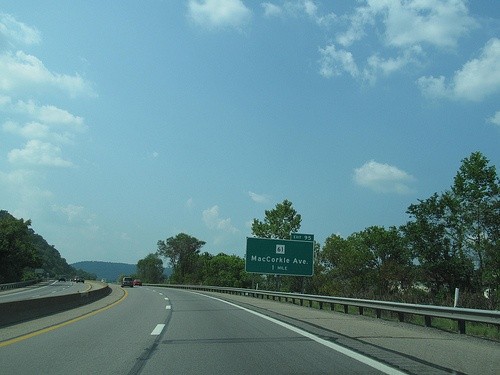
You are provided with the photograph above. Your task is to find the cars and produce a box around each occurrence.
[134,279,142,286]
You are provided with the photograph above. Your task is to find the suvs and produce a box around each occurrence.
[58,275,66,281]
[76,275,84,283]
[121,277,133,288]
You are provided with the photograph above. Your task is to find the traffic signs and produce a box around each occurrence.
[245,233,315,277]
